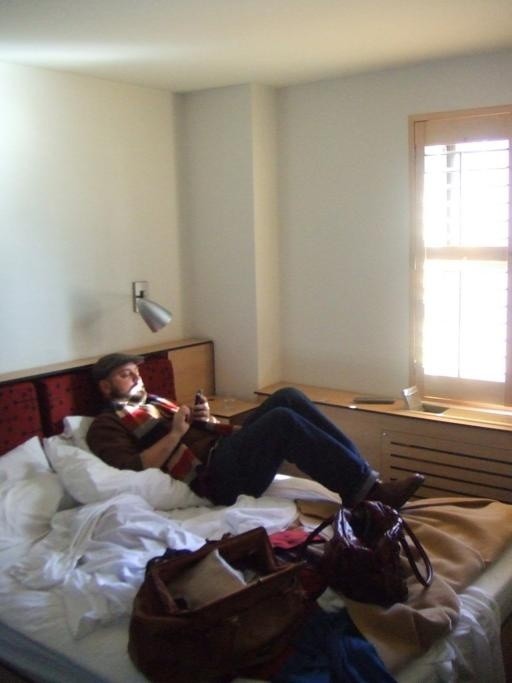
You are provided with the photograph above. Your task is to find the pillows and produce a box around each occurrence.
[0,414,215,515]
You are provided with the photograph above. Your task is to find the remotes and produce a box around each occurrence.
[353,395,396,404]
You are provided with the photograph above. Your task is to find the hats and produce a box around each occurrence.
[92,352,146,381]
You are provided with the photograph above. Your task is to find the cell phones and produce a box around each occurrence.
[195,389,202,404]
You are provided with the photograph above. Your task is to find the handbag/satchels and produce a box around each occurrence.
[288,499,434,608]
[127,525,316,683]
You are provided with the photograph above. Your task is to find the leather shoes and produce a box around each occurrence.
[364,472,425,510]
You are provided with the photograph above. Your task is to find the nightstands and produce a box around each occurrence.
[178,388,263,426]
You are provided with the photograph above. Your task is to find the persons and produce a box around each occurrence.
[86,353,425,510]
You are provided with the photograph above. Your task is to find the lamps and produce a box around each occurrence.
[133,280,175,332]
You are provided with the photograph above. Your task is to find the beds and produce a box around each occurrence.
[0,337,512,680]
